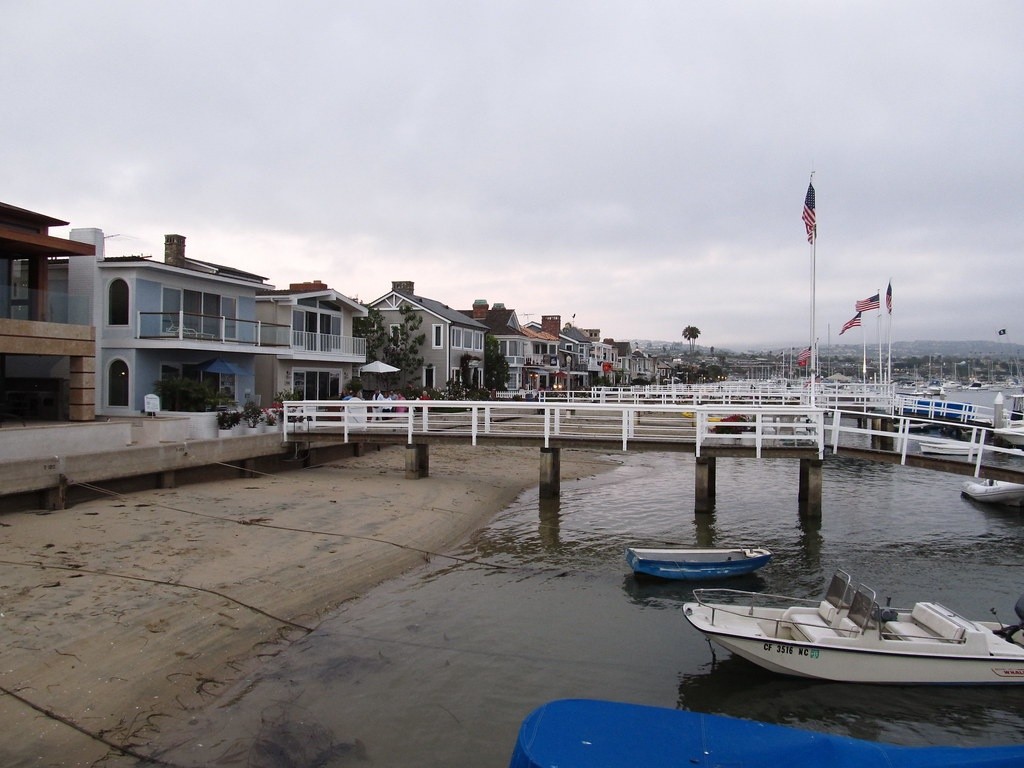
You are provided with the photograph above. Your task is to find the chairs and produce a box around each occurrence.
[797,601,858,648]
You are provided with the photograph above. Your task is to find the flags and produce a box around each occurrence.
[796,347,811,363]
[838,311,862,335]
[886,282,892,316]
[855,293,880,312]
[801,182,818,246]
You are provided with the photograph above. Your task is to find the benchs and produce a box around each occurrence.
[886,602,965,644]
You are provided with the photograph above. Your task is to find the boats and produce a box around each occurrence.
[627,542,776,582]
[506,695,1024,768]
[959,475,1024,504]
[879,360,1024,451]
[681,568,1024,685]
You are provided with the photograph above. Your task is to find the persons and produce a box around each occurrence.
[339,381,433,430]
[536,383,553,397]
[749,384,756,393]
